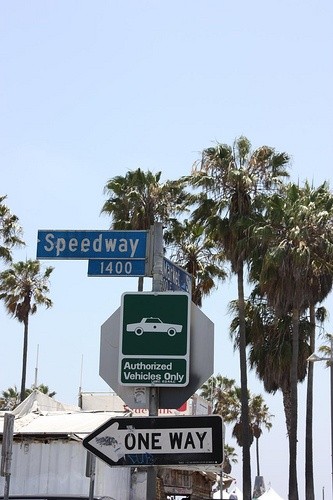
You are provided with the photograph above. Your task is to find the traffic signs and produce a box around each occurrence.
[162,256,192,293]
[35,229,146,277]
[82,415,222,468]
